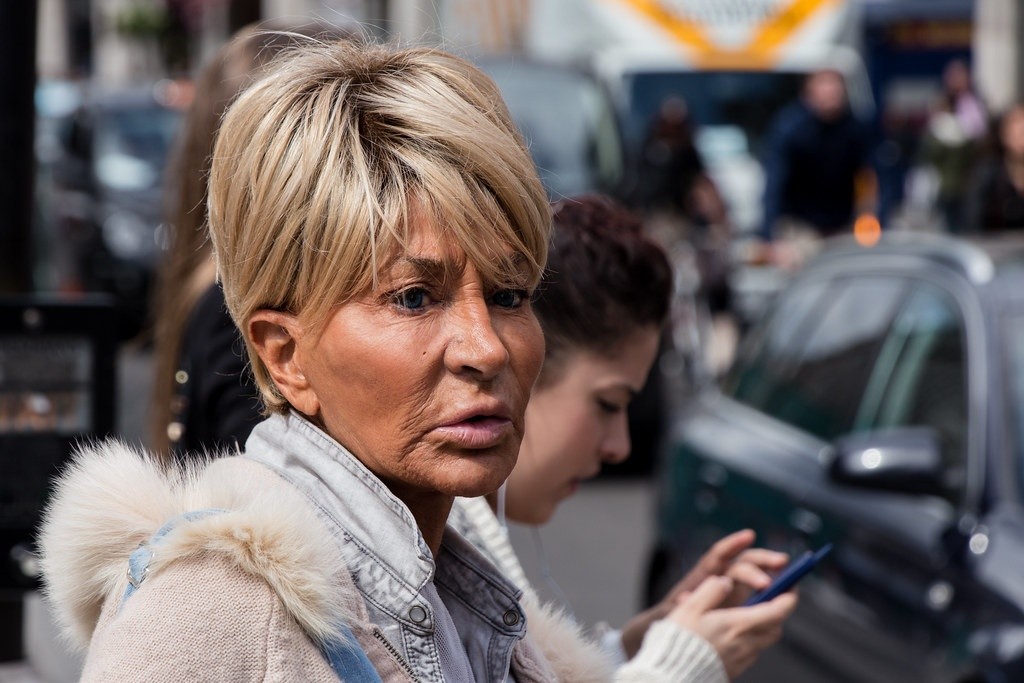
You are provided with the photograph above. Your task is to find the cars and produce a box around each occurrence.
[458,55,636,208]
[642,232,1024,683]
[28,82,192,297]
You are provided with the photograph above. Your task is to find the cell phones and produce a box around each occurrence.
[745,551,814,607]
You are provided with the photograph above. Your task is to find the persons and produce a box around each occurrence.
[445,195,799,683]
[923,59,1024,234]
[637,93,725,264]
[37,19,558,683]
[771,67,869,253]
